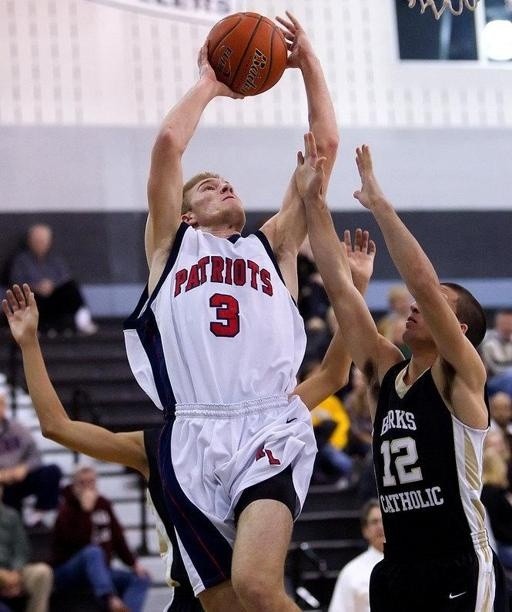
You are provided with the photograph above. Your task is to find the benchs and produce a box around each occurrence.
[13,317,168,428]
[292,482,368,604]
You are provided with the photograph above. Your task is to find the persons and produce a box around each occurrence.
[123,11,341,612]
[2,228,377,611]
[329,499,387,612]
[481,311,511,555]
[297,236,416,485]
[296,133,511,612]
[2,393,152,612]
[13,225,98,335]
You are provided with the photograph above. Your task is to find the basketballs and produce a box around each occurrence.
[206,13,289,95]
